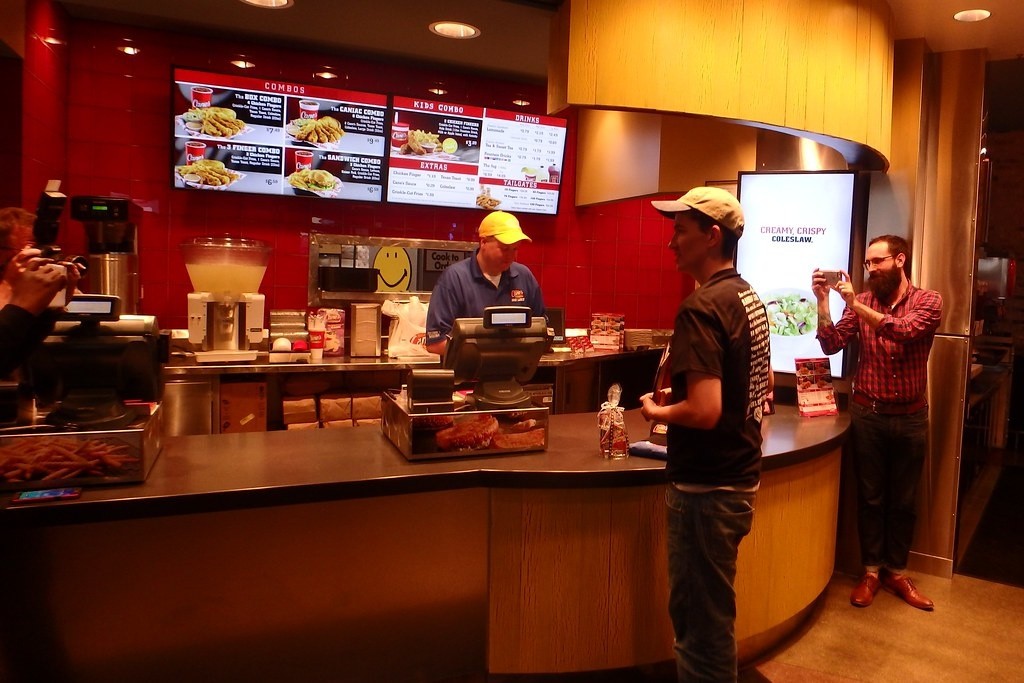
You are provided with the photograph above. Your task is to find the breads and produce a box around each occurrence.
[495,427,544,448]
[436,414,498,450]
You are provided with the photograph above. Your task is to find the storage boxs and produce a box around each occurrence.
[351,389,383,418]
[523,382,555,415]
[347,370,402,391]
[321,419,353,428]
[355,418,383,428]
[287,422,319,429]
[284,373,342,394]
[220,371,268,434]
[320,390,353,421]
[280,395,318,423]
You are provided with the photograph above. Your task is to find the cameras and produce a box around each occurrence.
[28,179,88,276]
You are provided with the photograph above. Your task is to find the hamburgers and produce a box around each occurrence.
[308,170,336,190]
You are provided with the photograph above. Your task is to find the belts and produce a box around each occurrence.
[852,390,928,416]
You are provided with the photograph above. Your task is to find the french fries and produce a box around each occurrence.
[1,436,139,481]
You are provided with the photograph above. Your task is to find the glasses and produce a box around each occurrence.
[864,254,896,271]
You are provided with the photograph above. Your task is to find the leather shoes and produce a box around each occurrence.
[883,574,934,609]
[850,572,882,608]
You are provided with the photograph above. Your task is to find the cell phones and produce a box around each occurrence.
[11,487,82,504]
[817,271,842,286]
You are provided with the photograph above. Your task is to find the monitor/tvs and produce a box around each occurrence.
[22,315,163,430]
[444,317,548,407]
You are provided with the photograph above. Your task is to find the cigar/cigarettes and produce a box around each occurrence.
[56,261,73,266]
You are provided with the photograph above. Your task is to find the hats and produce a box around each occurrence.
[478,211,532,245]
[651,186,745,240]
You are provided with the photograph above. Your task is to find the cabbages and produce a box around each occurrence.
[767,297,816,335]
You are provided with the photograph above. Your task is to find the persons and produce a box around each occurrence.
[813,236,943,609]
[639,188,774,683]
[425,212,546,370]
[0,208,82,380]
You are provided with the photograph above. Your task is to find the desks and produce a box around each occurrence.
[164,349,662,436]
